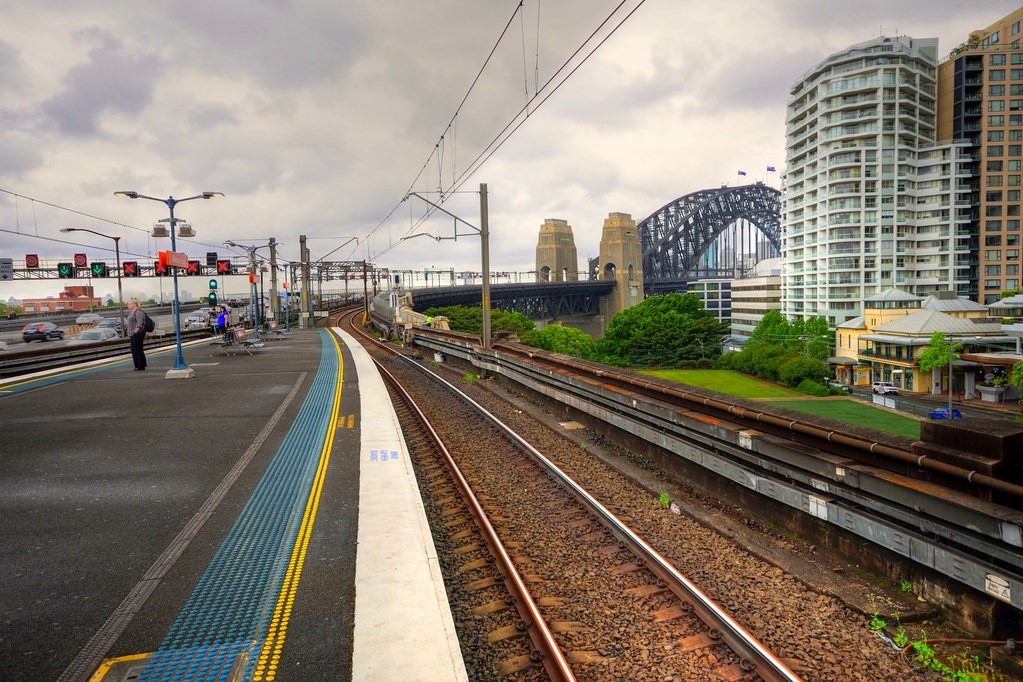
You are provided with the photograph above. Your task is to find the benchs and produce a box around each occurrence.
[209,328,263,356]
[248,321,287,342]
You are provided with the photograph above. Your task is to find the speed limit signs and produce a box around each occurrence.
[75,256,85,265]
[26,256,37,266]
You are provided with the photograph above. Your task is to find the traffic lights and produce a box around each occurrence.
[209,279,216,289]
[209,292,217,307]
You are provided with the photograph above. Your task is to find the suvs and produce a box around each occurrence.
[67,328,119,346]
[872,382,898,395]
[22,322,64,343]
[94,317,128,336]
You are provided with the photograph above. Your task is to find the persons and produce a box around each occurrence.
[217,306,229,336]
[128,299,147,370]
[426,315,431,327]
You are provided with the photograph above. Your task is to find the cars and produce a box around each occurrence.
[928,407,962,421]
[227,292,364,322]
[184,304,231,331]
[75,314,104,326]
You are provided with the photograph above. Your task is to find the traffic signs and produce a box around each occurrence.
[0,258,13,281]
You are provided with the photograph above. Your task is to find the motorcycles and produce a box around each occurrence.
[8,314,19,321]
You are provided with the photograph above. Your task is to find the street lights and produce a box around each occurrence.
[60,228,124,338]
[807,335,827,354]
[948,336,982,420]
[261,262,305,336]
[113,190,225,379]
[222,241,283,349]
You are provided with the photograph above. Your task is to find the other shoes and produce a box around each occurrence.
[134,367,145,370]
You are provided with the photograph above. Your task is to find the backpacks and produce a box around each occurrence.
[134,309,155,332]
